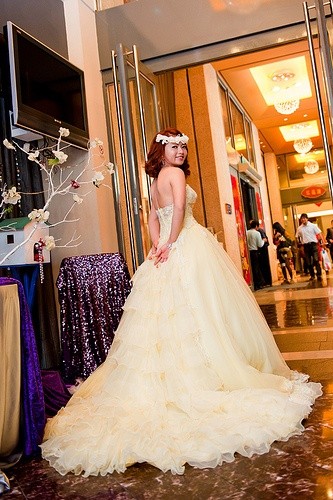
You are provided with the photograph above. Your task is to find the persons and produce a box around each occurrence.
[246,219,272,289]
[38,125,323,474]
[272,222,295,285]
[295,213,333,281]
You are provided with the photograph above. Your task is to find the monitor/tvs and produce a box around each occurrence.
[2,21,89,151]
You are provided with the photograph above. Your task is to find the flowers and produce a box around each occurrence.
[0,128,122,271]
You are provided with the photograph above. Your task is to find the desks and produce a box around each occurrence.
[0,277,45,459]
[56,253,129,381]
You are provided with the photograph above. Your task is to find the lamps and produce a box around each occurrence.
[269,72,301,116]
[288,124,314,153]
[296,152,320,175]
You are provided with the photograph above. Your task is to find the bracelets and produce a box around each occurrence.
[166,243,172,248]
